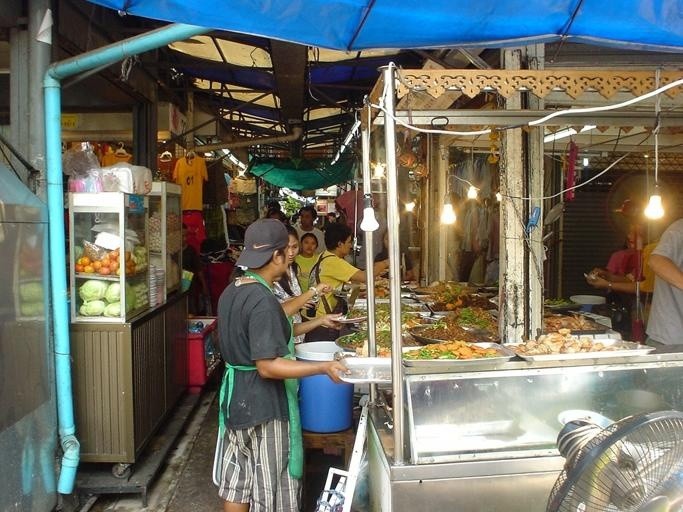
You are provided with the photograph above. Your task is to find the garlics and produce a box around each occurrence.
[133,282,148,308]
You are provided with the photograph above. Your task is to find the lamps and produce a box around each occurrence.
[441,171,456,224]
[467,146,478,199]
[360,99,379,231]
[644,128,666,218]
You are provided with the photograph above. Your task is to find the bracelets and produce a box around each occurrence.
[308,286,321,303]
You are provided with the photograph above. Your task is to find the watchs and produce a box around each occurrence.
[607,280,616,294]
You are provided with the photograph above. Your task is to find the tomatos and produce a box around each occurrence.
[75,252,135,277]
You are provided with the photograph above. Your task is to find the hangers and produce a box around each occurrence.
[160,141,173,161]
[186,143,194,157]
[115,141,131,159]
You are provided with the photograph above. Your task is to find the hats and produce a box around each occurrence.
[234,219,288,269]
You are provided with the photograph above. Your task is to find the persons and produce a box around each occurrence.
[212,219,352,512]
[293,205,329,255]
[299,221,404,344]
[294,233,323,295]
[272,224,346,360]
[374,227,414,281]
[608,246,644,345]
[265,199,299,239]
[582,239,658,345]
[642,215,683,355]
[321,213,338,232]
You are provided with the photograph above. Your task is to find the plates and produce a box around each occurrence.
[330,313,369,323]
[360,313,438,333]
[334,331,422,355]
[570,294,607,309]
[405,323,500,347]
[334,284,368,297]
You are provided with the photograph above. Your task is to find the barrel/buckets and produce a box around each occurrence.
[569,294,606,313]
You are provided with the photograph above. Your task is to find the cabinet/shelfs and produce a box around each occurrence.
[148,182,184,303]
[67,192,153,325]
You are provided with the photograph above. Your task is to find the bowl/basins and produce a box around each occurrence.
[147,264,164,307]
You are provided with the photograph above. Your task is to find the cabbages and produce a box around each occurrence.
[77,281,133,317]
[19,282,44,316]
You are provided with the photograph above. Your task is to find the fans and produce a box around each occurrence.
[545,408,683,512]
[608,171,680,347]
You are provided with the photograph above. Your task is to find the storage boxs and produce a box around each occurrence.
[188,316,221,392]
